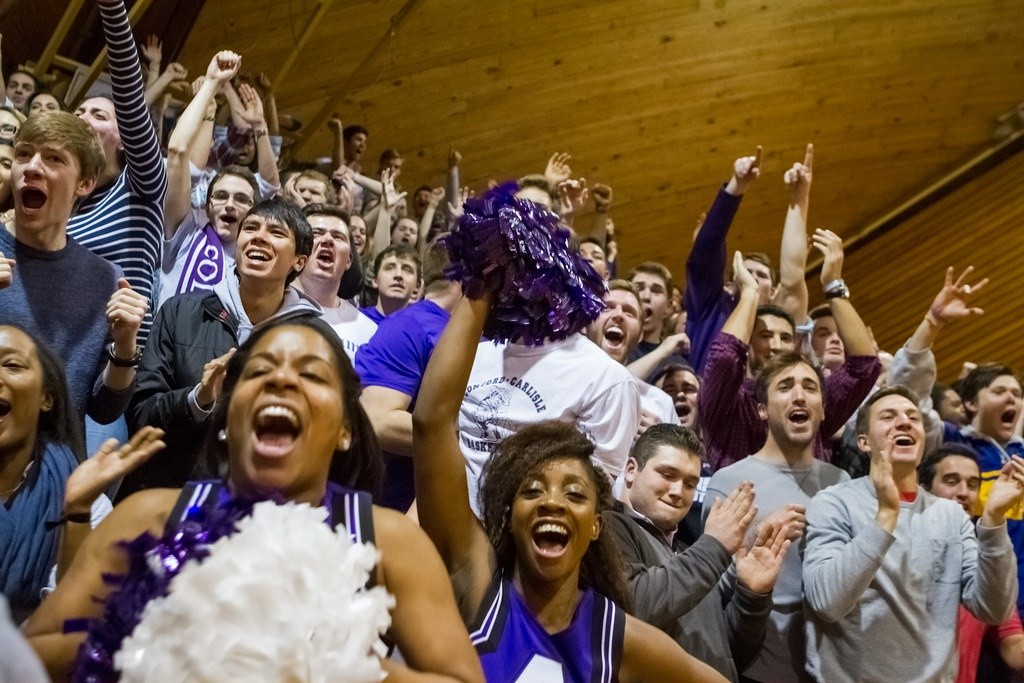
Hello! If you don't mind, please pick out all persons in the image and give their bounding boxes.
[0,0,1024,683]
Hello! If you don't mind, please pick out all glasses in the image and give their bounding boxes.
[1,124,17,135]
[211,192,254,206]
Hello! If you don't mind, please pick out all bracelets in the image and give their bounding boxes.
[203,115,215,121]
[254,129,268,135]
[823,278,844,292]
[104,341,141,367]
[44,511,91,531]
[925,313,943,330]
[57,552,77,569]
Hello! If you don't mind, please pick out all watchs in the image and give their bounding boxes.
[825,284,850,299]
